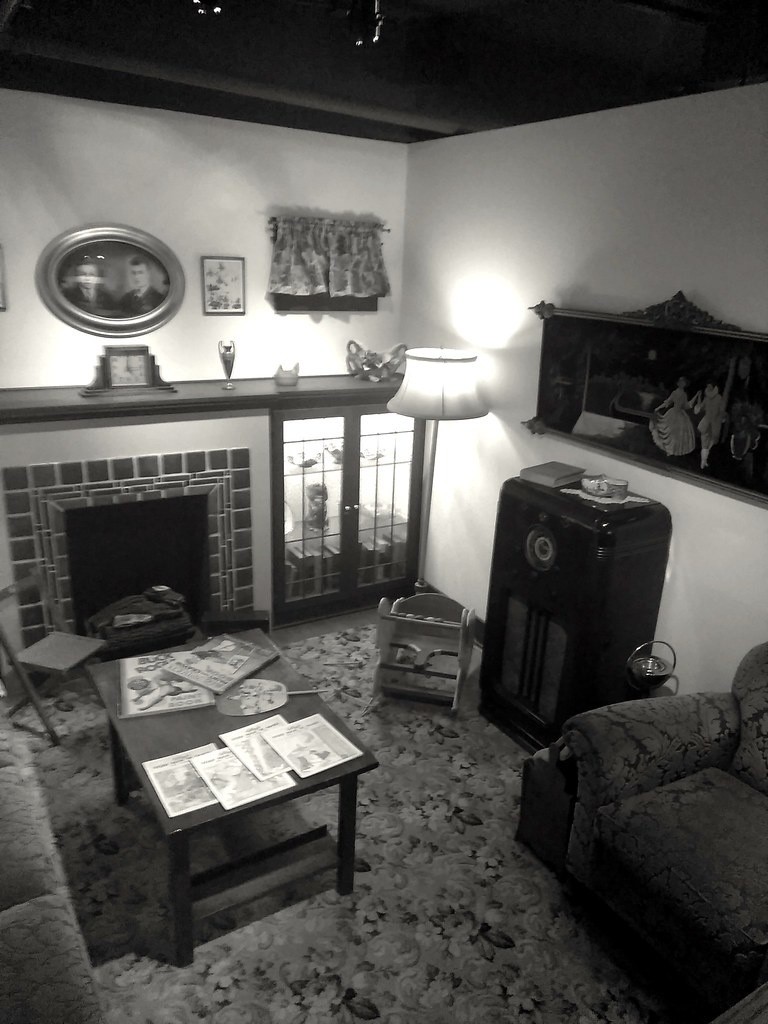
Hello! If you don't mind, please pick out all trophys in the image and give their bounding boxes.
[219,341,236,389]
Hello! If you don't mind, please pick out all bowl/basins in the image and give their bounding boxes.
[582,474,628,499]
[324,442,344,464]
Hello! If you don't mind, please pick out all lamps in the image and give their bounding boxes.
[344,0,384,45]
[386,346,490,598]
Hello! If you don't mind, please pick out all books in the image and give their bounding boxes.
[142,713,363,818]
[116,632,280,718]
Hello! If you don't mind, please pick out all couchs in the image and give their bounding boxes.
[0,742,103,1024]
[512,644,768,1024]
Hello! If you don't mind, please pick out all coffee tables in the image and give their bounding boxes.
[82,628,380,969]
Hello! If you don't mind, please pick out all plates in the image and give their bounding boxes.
[360,448,385,460]
[289,453,322,467]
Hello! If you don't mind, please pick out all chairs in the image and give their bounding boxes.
[0,567,106,745]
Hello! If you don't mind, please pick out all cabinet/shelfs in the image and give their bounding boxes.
[476,477,673,756]
[271,402,426,630]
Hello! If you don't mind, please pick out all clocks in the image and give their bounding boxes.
[79,345,180,396]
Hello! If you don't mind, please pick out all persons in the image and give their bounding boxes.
[66,256,163,318]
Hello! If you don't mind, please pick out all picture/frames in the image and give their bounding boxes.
[522,290,768,511]
[35,222,186,339]
[201,255,246,315]
